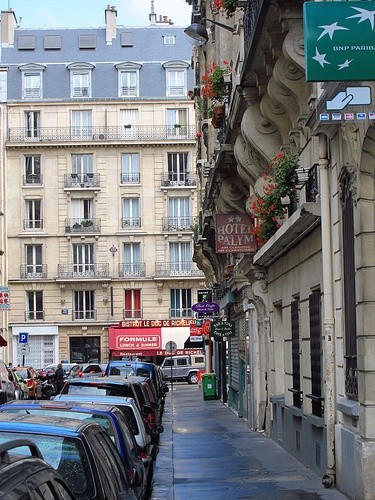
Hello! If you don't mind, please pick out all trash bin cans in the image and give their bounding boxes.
[201,373,217,401]
[198,370,206,388]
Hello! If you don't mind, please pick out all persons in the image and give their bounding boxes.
[55,364,66,394]
[7,363,12,368]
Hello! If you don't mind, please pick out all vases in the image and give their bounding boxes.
[238,0,247,7]
[280,195,291,204]
[222,73,232,82]
[294,168,309,185]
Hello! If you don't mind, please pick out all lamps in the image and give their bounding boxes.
[183,18,237,46]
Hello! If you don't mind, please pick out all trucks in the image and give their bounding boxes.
[160,355,205,384]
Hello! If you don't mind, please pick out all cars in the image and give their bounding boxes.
[0,439,80,500]
[0,361,169,500]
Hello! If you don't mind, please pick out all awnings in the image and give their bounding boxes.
[109,327,203,356]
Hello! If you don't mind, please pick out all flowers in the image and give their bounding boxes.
[200,59,228,98]
[213,0,240,19]
[249,153,297,250]
